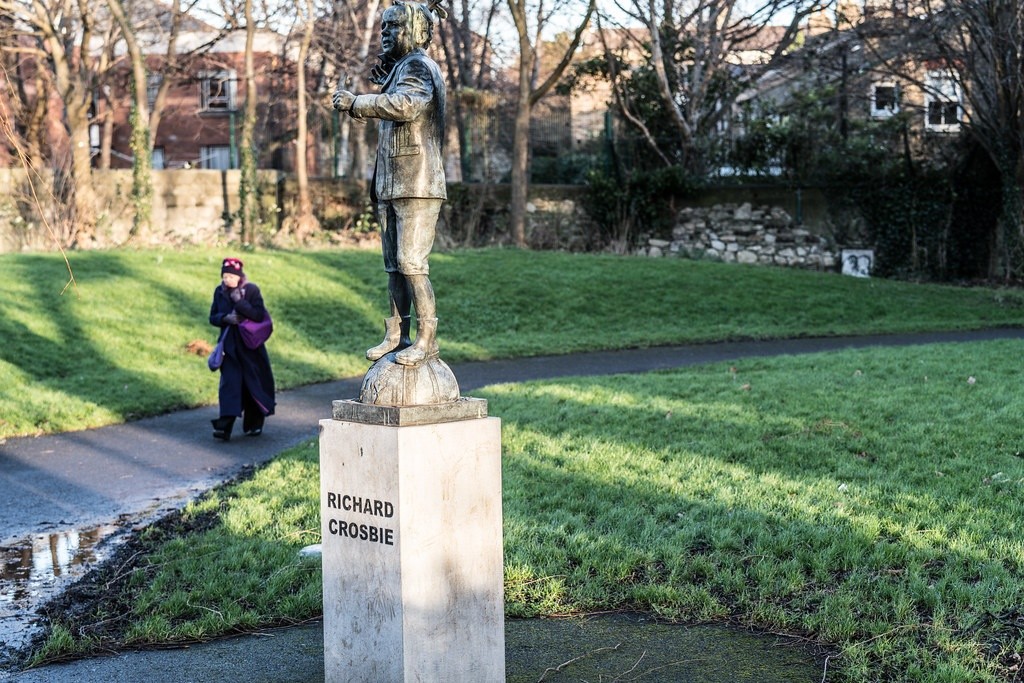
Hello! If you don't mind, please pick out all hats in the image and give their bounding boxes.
[221,258,244,276]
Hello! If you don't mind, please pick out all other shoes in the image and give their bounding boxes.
[213,431,231,441]
[246,430,262,437]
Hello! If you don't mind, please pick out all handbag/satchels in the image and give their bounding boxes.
[241,310,273,350]
[207,342,225,371]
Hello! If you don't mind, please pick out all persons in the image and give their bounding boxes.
[209,257,277,440]
[332,0,447,367]
